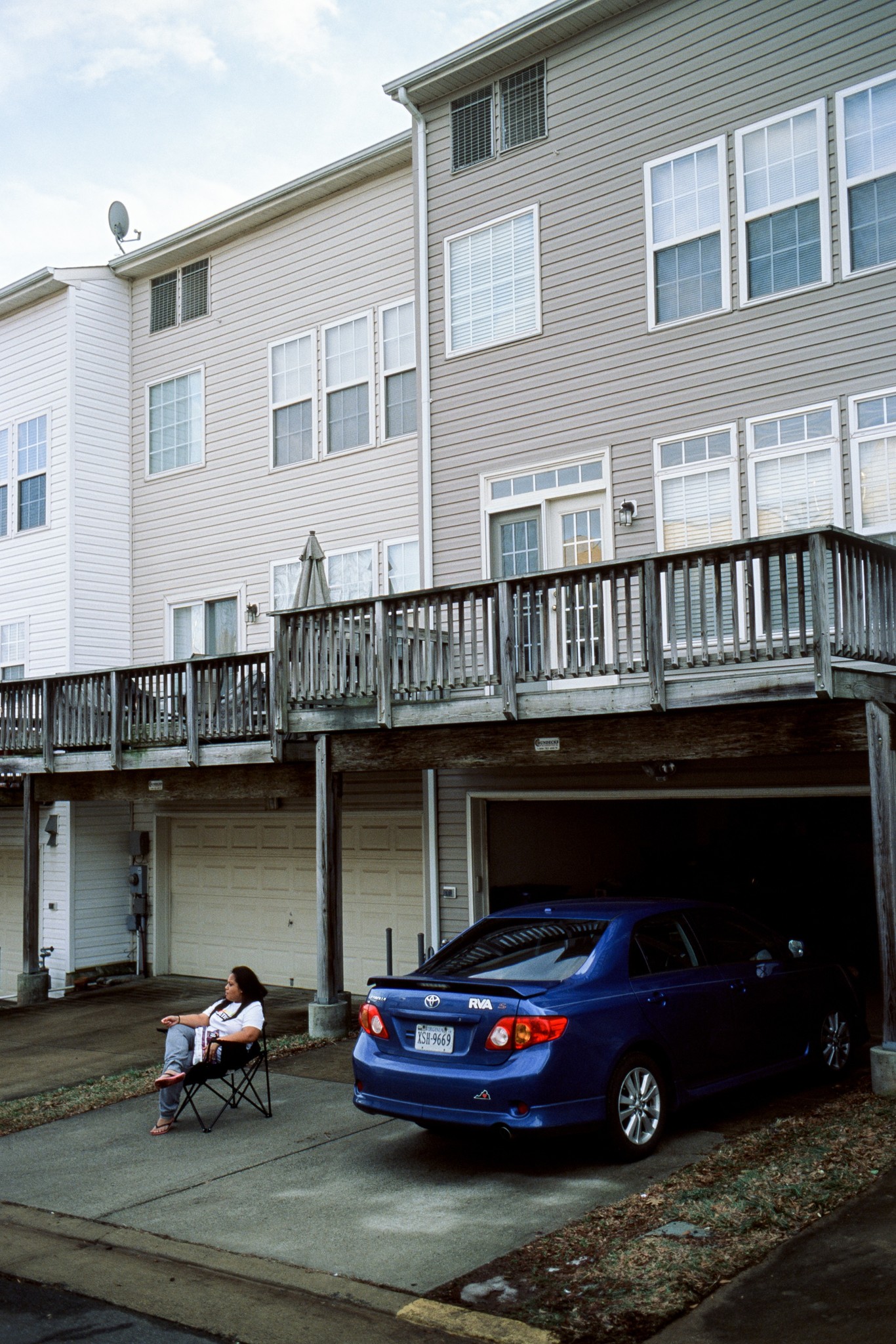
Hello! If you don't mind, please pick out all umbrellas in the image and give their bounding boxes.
[292,531,331,608]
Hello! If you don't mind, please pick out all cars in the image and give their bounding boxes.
[352,894,866,1163]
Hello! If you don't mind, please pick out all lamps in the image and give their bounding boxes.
[618,499,638,525]
[245,602,259,623]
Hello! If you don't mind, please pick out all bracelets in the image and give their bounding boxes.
[178,1015,180,1023]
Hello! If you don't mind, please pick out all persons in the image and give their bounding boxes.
[150,966,268,1135]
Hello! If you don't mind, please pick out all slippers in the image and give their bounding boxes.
[151,1116,174,1134]
[155,1070,185,1088]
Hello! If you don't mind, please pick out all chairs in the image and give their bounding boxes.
[157,1022,272,1133]
